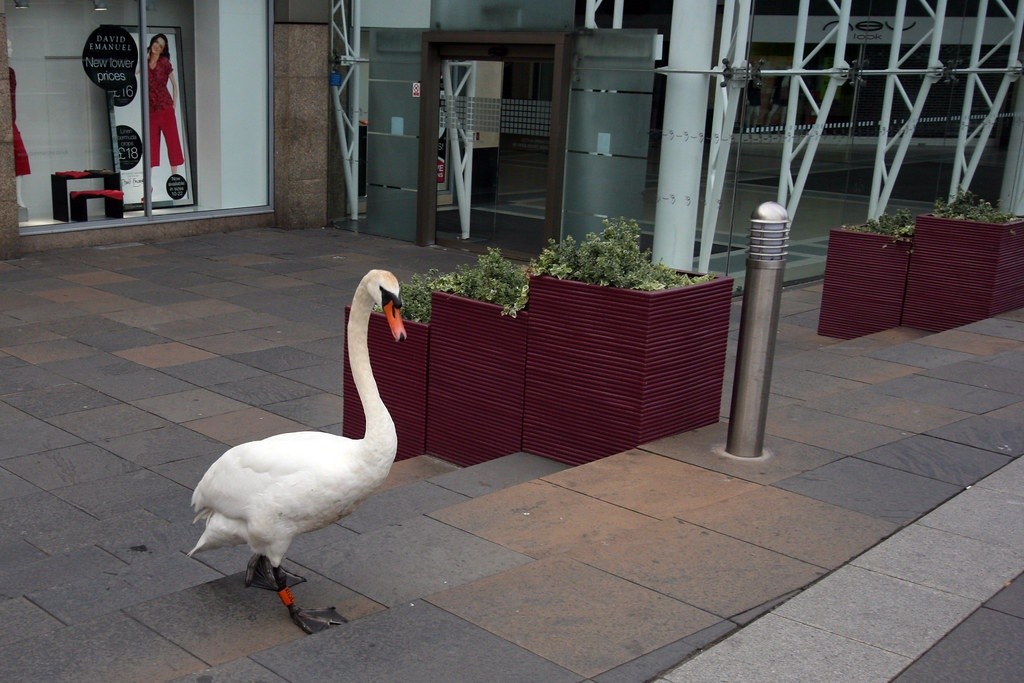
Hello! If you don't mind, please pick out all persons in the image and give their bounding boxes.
[9,66,31,210]
[141,32,185,201]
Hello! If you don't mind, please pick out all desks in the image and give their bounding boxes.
[51,173,120,222]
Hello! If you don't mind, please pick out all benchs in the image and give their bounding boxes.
[69,190,124,222]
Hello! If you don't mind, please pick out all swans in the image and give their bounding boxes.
[188,270,410,637]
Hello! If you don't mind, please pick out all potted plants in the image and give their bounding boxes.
[816,184,1024,340]
[342,215,734,467]
